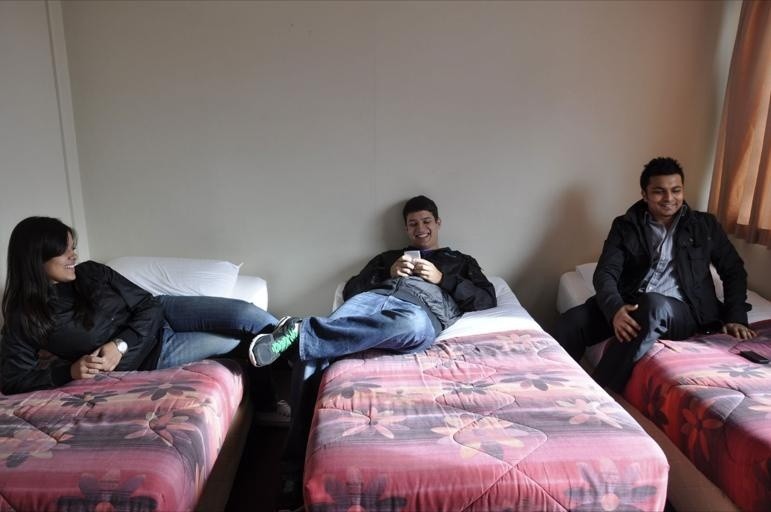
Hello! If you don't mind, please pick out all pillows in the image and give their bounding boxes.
[104,258,244,299]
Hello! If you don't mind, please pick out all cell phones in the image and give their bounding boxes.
[404,250,420,259]
[740,350,769,363]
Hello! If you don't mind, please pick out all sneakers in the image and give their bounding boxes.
[249,316,303,367]
[279,473,303,512]
[254,400,292,422]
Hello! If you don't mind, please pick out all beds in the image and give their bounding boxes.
[559,260,771,512]
[0,275,269,511]
[304,262,670,511]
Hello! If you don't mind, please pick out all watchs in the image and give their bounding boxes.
[115,338,129,355]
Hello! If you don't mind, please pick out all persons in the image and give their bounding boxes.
[248,195,498,511]
[0,216,294,427]
[552,155,760,394]
